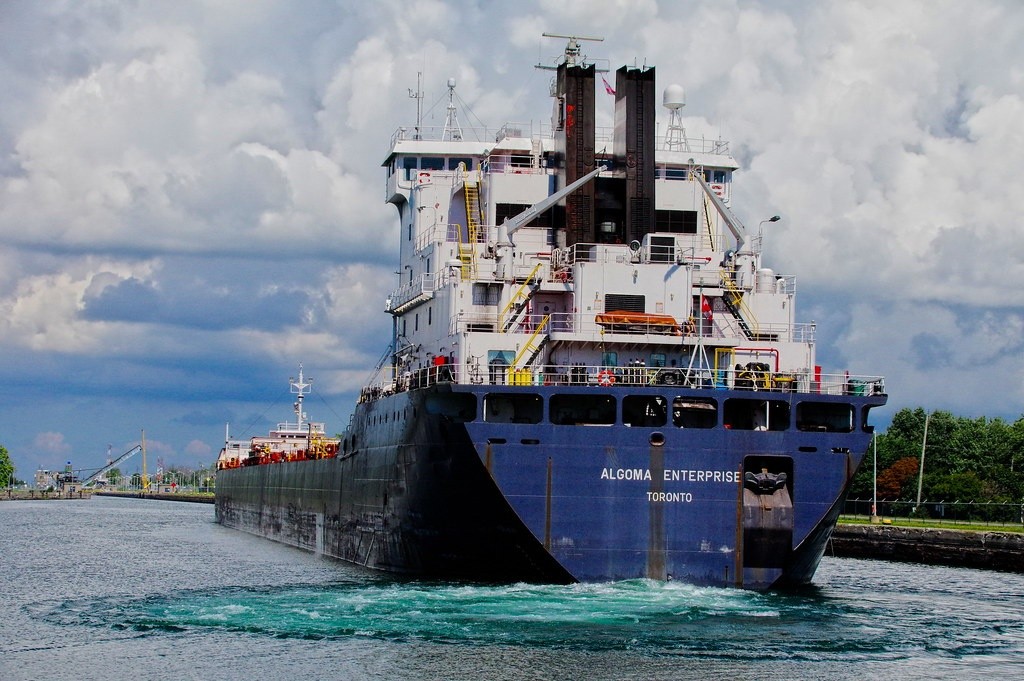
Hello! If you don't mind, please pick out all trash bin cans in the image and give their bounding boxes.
[848,379,865,396]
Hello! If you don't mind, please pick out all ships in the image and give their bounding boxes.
[214,30,892,603]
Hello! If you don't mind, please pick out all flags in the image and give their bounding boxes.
[702,294,713,325]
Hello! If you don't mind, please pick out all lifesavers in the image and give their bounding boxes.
[598,369,615,387]
[419,172,431,184]
[712,184,722,196]
